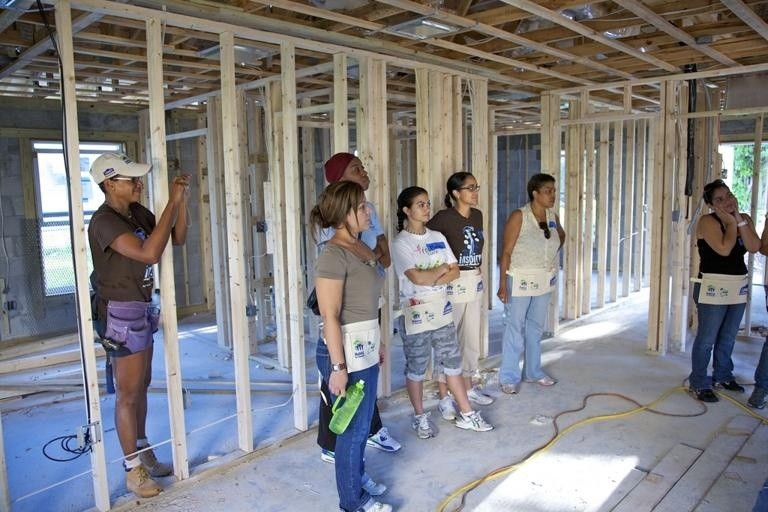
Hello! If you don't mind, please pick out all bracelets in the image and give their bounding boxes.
[737,219,749,227]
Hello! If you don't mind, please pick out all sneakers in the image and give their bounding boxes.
[689,385,717,402]
[366,427,402,452]
[126,463,161,497]
[414,385,494,439]
[355,497,392,512]
[321,449,365,463]
[362,478,386,495]
[138,445,172,476]
[712,380,744,392]
[522,375,555,386]
[747,387,768,409]
[503,384,517,394]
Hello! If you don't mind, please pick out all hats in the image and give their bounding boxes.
[325,153,354,183]
[89,151,152,184]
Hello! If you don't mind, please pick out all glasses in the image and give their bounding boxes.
[539,222,549,238]
[704,179,723,201]
[112,178,139,182]
[457,186,480,191]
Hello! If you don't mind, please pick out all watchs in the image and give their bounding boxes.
[331,363,346,371]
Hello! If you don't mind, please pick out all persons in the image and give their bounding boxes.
[746,211,768,409]
[309,180,393,512]
[393,186,494,438]
[316,152,402,463]
[688,179,761,402]
[497,173,566,395]
[87,151,193,497]
[424,172,494,420]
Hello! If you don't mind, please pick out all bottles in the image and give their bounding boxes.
[501,299,512,326]
[328,379,365,435]
